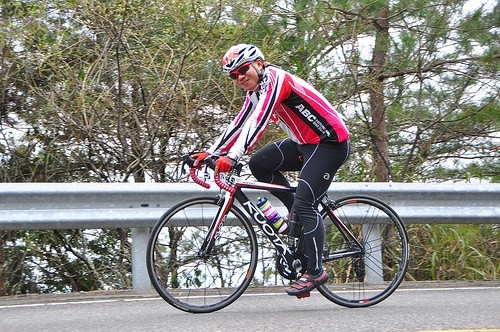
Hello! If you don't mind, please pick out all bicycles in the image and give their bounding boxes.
[147,152,410,312]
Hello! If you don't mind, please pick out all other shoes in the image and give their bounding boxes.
[285,268,328,294]
[283,213,301,236]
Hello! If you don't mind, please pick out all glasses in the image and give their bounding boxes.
[229,61,261,80]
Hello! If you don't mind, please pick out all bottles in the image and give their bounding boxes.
[256,196,288,233]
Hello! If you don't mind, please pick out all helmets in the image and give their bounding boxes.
[221,43,263,72]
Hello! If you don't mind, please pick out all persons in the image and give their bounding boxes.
[190,44,350,295]
